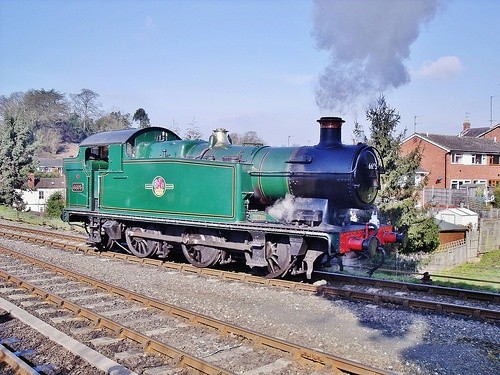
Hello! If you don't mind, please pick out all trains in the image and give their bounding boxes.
[63,116,404,280]
